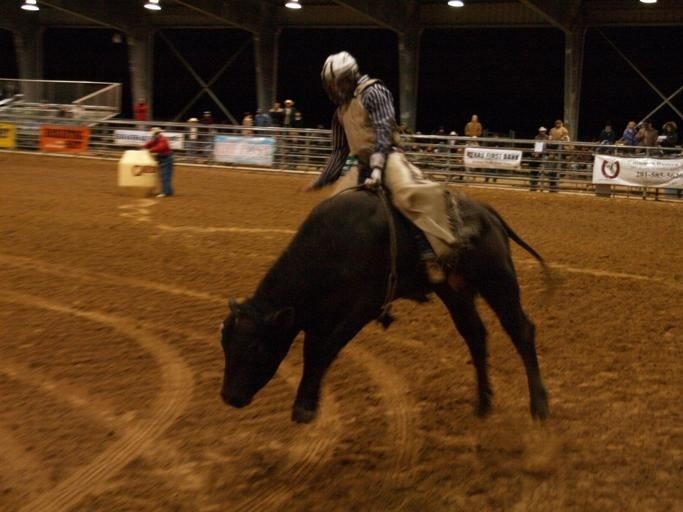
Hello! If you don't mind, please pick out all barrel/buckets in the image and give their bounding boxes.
[117,150,160,187]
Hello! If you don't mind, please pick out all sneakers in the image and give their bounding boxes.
[425,259,446,284]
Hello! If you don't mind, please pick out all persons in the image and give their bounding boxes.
[199,110,213,156]
[185,117,200,164]
[394,113,678,194]
[133,97,151,131]
[134,127,173,198]
[294,51,447,284]
[241,98,307,171]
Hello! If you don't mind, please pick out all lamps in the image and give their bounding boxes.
[19,0,40,13]
[283,0,303,11]
[143,0,162,13]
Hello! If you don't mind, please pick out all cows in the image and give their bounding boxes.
[219,186,569,426]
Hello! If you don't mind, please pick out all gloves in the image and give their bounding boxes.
[365,168,383,189]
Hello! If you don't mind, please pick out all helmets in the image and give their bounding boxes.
[320,50,360,86]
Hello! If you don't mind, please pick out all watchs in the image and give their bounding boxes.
[7,93,83,154]
